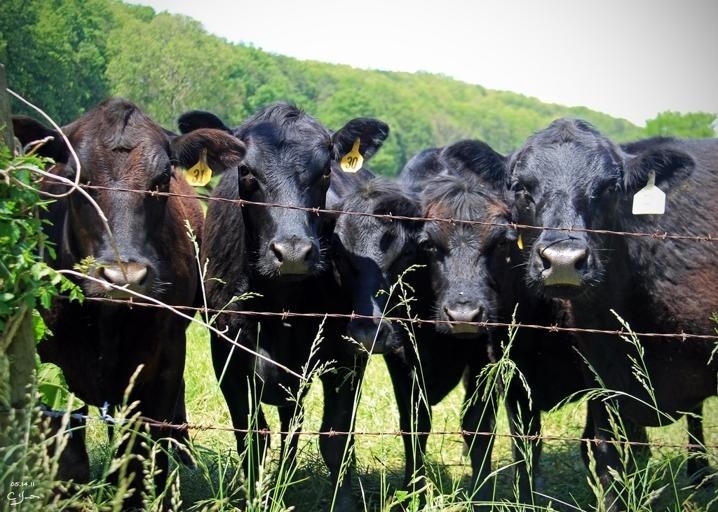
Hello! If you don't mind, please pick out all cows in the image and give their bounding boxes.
[311,174,433,512]
[434,118,718,512]
[398,146,541,512]
[10,97,248,512]
[175,100,390,512]
